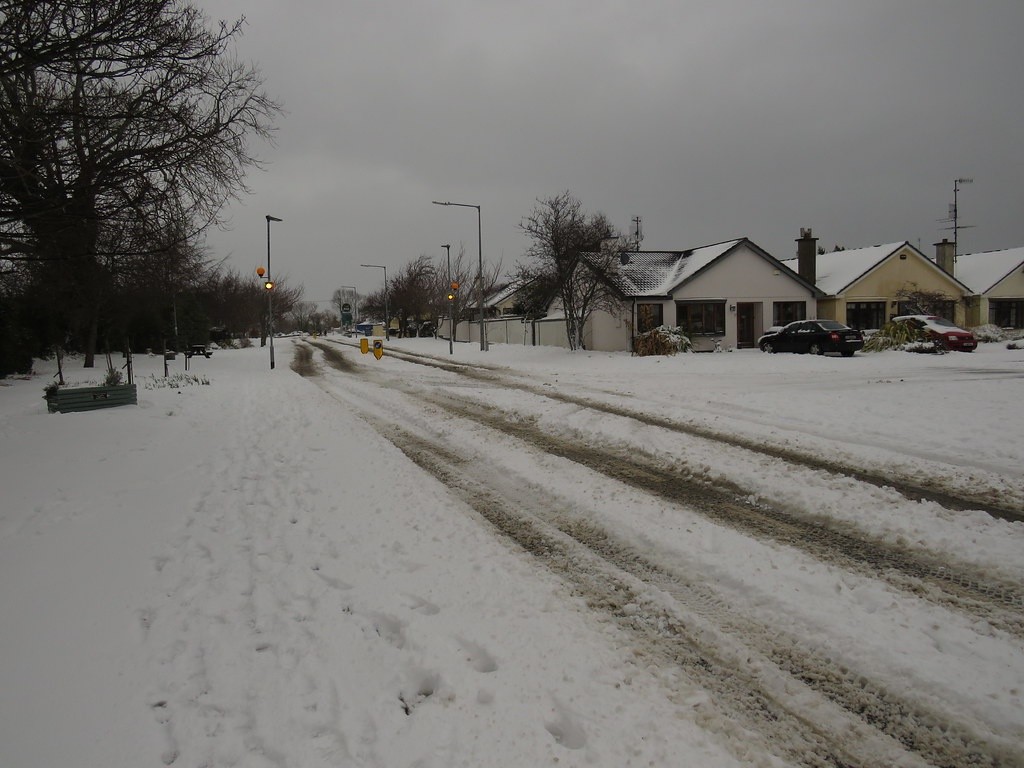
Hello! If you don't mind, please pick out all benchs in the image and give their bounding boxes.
[184,345,213,359]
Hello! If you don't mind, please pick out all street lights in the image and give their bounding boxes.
[257,215,284,370]
[361,265,389,341]
[348,287,358,338]
[431,200,486,352]
[441,244,460,353]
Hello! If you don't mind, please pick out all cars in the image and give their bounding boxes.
[757,318,864,359]
[892,314,978,352]
